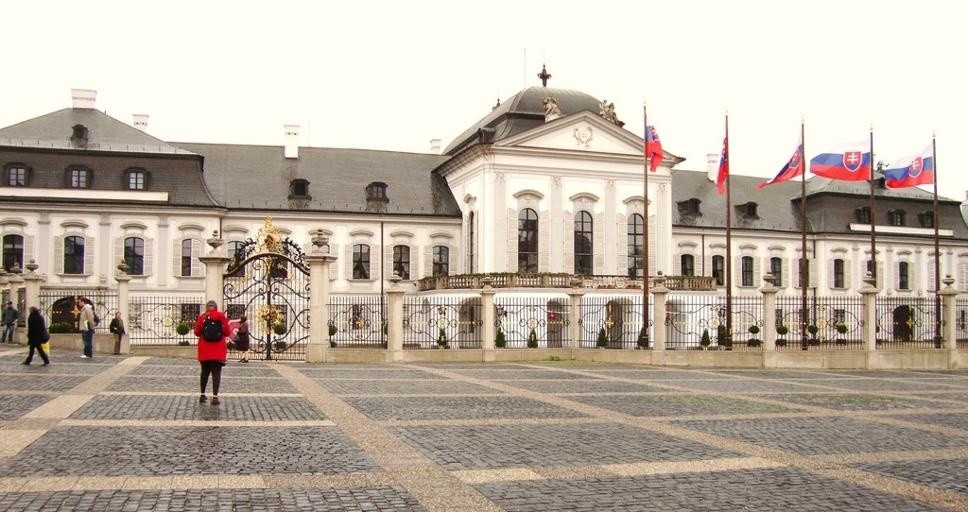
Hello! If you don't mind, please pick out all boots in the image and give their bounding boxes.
[199,395,221,405]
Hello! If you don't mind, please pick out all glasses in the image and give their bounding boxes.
[6,301,13,304]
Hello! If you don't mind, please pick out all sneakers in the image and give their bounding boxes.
[238,358,249,363]
[41,362,49,366]
[22,361,29,365]
[79,353,91,359]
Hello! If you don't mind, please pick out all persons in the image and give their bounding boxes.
[110,312,127,355]
[234,316,249,363]
[0,301,18,344]
[194,300,231,405]
[543,94,561,116]
[599,99,625,126]
[21,307,49,366]
[79,297,95,359]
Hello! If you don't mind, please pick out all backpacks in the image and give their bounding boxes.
[201,319,223,341]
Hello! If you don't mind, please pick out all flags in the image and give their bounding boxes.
[646,126,663,172]
[810,151,871,181]
[883,156,934,188]
[756,144,802,188]
[718,138,728,195]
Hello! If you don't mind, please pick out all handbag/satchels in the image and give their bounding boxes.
[39,327,49,343]
